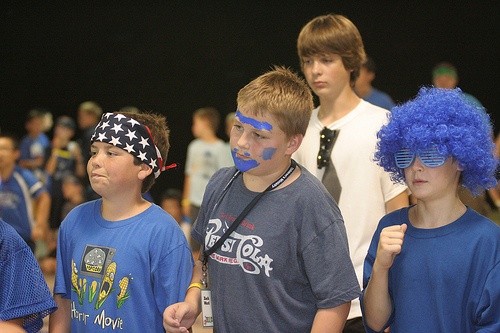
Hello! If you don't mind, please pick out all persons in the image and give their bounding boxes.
[48,113,195,333]
[1,14,500,333]
[359,85,500,333]
[1,219,58,333]
[162,66,362,333]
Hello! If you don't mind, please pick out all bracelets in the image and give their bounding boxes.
[186,282,204,290]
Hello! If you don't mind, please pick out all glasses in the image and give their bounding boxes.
[392,141,458,169]
